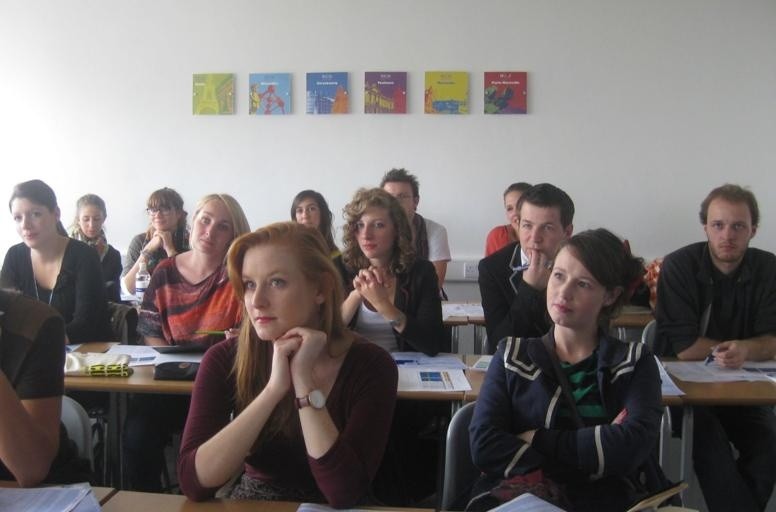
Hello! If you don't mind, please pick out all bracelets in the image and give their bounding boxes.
[139,249,151,260]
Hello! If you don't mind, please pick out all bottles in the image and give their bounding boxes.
[135,263,150,304]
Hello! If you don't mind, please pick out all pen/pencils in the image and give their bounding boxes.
[704,348,719,365]
[395,359,419,364]
[194,331,224,334]
[513,260,554,271]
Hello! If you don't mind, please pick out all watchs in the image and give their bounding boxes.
[294,389,328,410]
[386,312,405,328]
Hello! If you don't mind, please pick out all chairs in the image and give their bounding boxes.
[107,302,138,345]
[58,396,97,483]
[642,319,656,354]
[443,399,475,511]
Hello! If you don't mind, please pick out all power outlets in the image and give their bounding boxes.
[463,264,479,278]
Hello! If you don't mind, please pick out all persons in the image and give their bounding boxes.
[176,222,398,508]
[0,288,64,489]
[486,182,533,257]
[0,179,104,408]
[333,188,441,507]
[463,229,682,512]
[122,194,251,492]
[290,190,358,328]
[477,184,576,354]
[653,185,776,512]
[67,195,122,304]
[119,187,190,298]
[381,168,452,291]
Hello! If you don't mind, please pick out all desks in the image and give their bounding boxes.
[464,354,683,479]
[468,298,656,354]
[64,342,464,505]
[0,481,118,511]
[441,300,468,353]
[101,488,437,512]
[658,357,776,498]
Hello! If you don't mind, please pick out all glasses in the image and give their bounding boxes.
[147,207,178,216]
